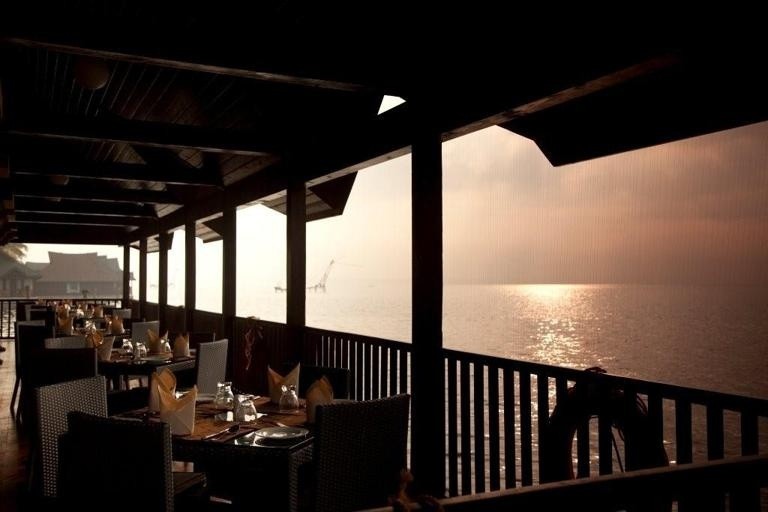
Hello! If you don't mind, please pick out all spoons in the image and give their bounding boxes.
[214,424,240,440]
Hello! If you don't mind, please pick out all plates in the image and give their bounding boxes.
[196,394,214,403]
[254,426,309,439]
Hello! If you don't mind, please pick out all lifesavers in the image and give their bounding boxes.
[540,384,669,482]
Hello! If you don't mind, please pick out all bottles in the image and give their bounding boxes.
[279,383,299,414]
[118,338,172,359]
[213,381,257,429]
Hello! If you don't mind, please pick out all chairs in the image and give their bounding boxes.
[9,298,410,510]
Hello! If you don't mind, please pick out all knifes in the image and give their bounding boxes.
[202,428,232,440]
